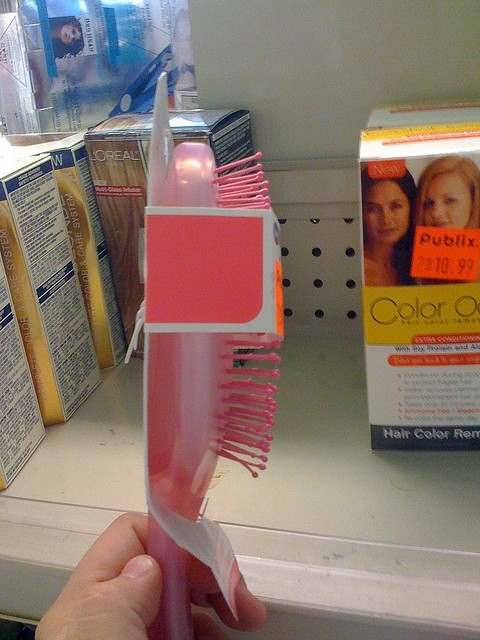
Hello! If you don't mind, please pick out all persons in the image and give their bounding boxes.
[412,156,479,232]
[360,157,419,284]
[34,509,268,640]
[46,15,85,60]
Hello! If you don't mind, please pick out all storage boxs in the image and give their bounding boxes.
[365,99,480,130]
[0,257,48,492]
[358,121,480,451]
[0,1,197,128]
[0,152,105,425]
[1,130,131,368]
[83,110,259,369]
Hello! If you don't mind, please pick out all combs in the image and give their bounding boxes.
[146,67,283,640]
